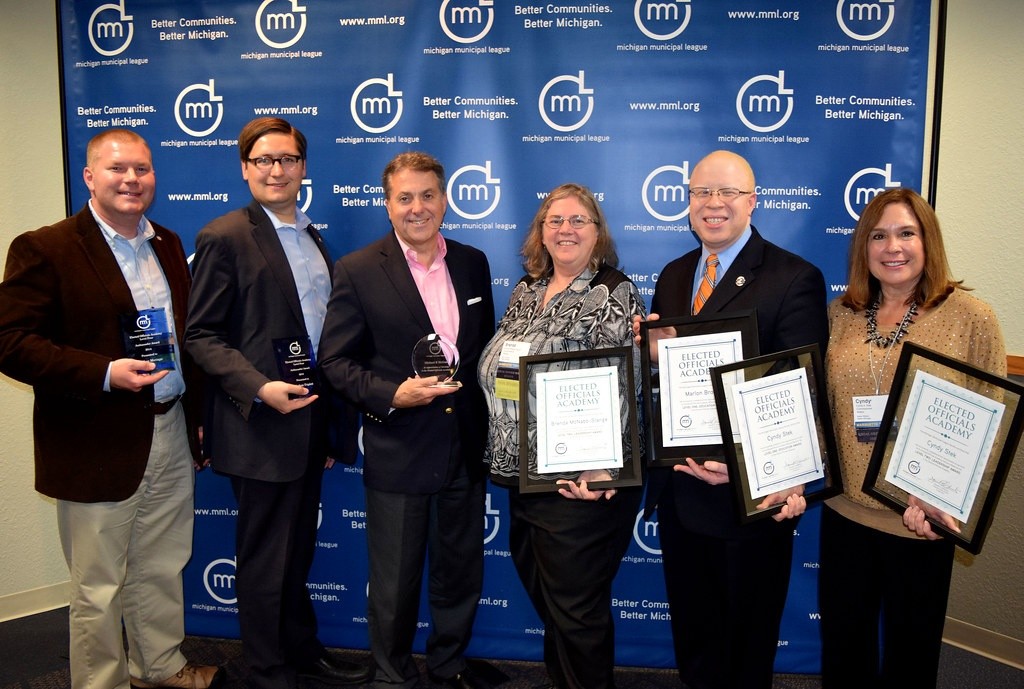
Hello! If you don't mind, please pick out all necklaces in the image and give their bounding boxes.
[868,305,912,397]
[862,289,919,350]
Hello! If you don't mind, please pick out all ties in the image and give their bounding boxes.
[693,256,719,316]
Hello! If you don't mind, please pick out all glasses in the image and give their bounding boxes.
[541,215,600,230]
[246,153,303,172]
[688,187,754,199]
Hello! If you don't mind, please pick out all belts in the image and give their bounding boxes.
[156,395,182,416]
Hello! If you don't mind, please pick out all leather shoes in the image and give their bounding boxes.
[428,662,490,689]
[294,648,373,684]
[129,663,216,689]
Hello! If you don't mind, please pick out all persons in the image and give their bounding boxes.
[632,149,833,689]
[184,116,373,689]
[316,152,500,687]
[472,182,652,689]
[0,125,220,689]
[820,181,1008,689]
